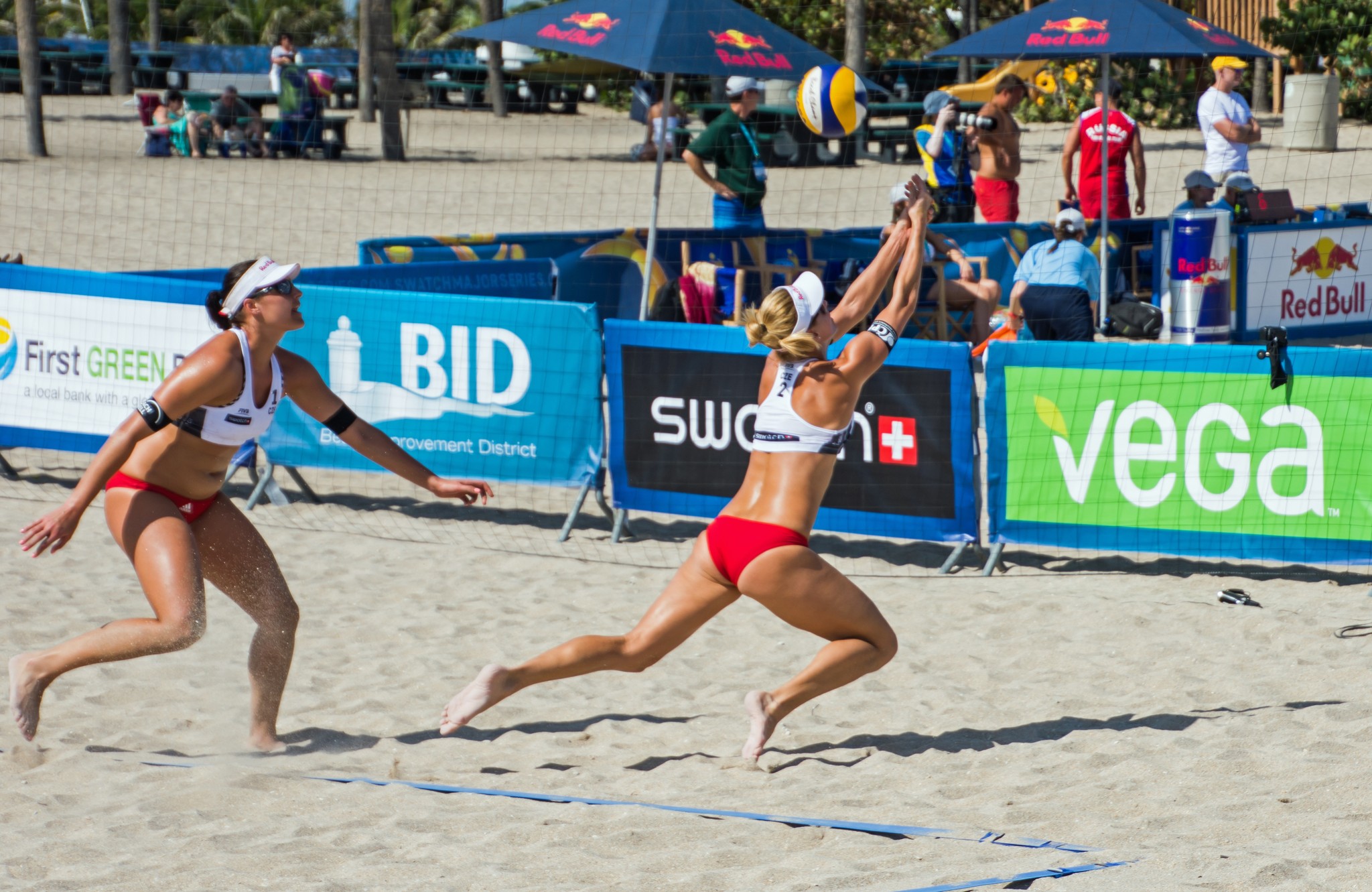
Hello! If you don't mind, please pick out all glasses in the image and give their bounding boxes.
[808,304,827,331]
[239,278,294,311]
[749,88,758,94]
[1232,68,1245,73]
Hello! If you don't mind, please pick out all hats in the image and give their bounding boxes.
[923,91,961,114]
[771,271,824,352]
[218,254,301,321]
[1225,170,1254,190]
[725,76,769,96]
[1182,170,1223,189]
[888,182,918,204]
[1054,207,1086,232]
[1211,56,1249,71]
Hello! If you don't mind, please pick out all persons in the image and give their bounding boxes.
[152,31,297,158]
[645,89,687,147]
[1197,54,1263,205]
[681,76,766,229]
[878,170,1262,342]
[437,174,941,766]
[913,90,981,224]
[973,74,1029,222]
[11,255,495,751]
[1062,78,1147,218]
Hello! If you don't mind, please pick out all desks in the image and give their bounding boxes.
[685,103,854,168]
[0,50,181,96]
[447,64,582,114]
[179,92,279,118]
[855,101,985,163]
[301,62,448,108]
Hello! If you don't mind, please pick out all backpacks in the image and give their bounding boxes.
[649,277,687,322]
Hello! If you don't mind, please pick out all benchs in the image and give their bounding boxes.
[874,130,921,163]
[329,83,378,107]
[234,115,352,145]
[79,69,114,95]
[0,68,21,93]
[134,66,195,90]
[666,128,785,168]
[424,80,521,113]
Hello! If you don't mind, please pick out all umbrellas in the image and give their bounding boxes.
[452,0,889,323]
[927,0,1277,334]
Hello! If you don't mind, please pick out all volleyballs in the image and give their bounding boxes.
[796,62,868,138]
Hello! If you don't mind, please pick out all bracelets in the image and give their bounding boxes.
[1007,312,1018,318]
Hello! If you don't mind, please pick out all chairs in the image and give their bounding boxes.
[1132,244,1153,298]
[134,92,212,158]
[682,241,772,327]
[760,237,867,333]
[884,257,989,341]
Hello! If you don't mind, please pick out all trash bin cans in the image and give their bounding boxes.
[1283,74,1342,151]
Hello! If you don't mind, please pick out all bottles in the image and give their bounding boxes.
[988,314,1007,331]
